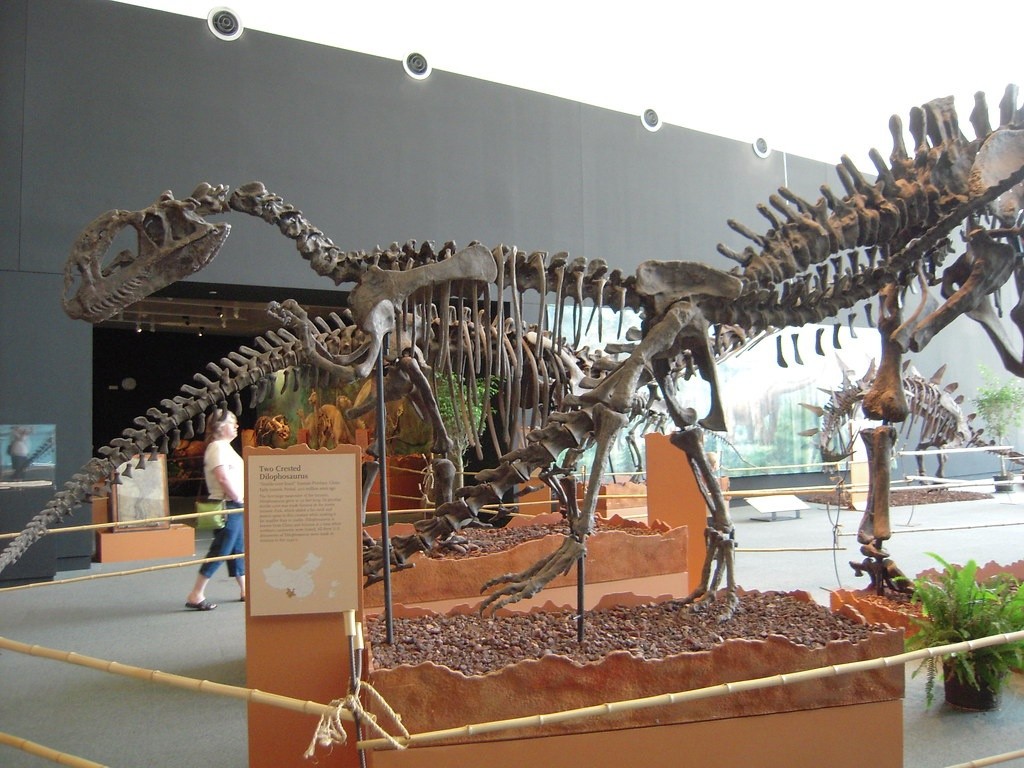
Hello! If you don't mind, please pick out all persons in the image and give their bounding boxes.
[184,409,245,611]
[698,451,720,485]
[253,416,286,448]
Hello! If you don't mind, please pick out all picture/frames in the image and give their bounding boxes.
[110,452,170,533]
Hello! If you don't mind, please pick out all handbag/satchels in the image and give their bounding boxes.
[193,501,226,531]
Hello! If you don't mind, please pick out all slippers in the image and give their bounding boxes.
[184,599,218,610]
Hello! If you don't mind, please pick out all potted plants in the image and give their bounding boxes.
[970,363,1024,492]
[891,551,1024,716]
